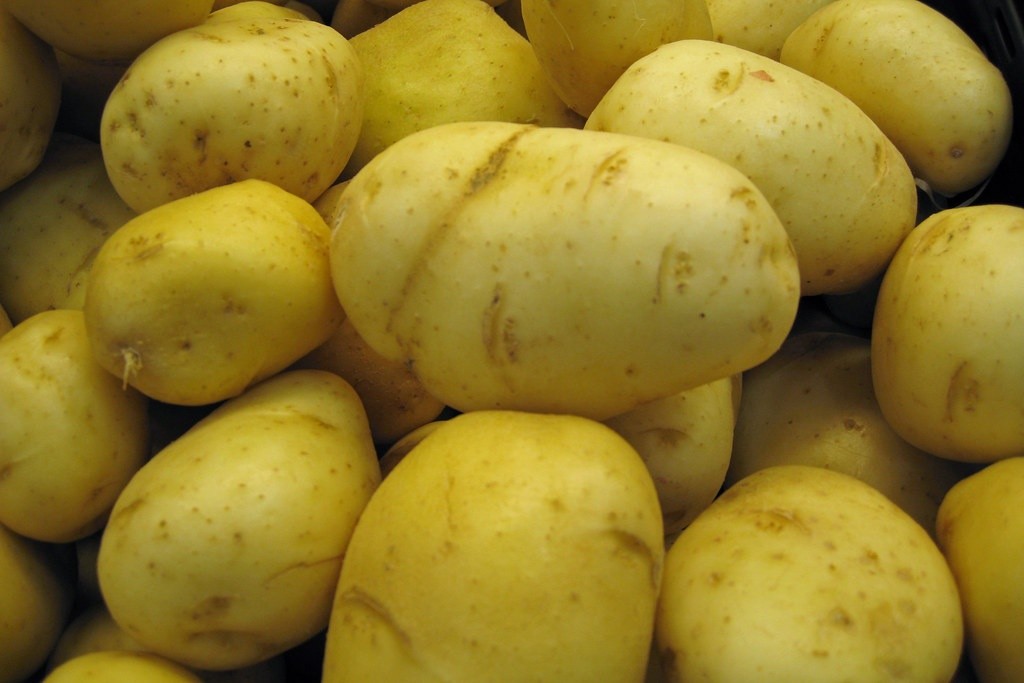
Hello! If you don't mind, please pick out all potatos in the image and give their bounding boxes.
[0,0,1024,683]
